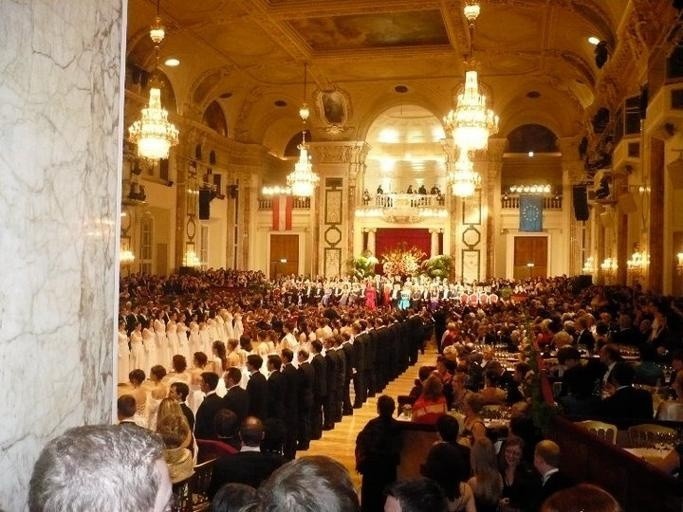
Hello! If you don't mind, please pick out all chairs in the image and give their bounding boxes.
[628,425,677,447]
[575,421,618,444]
[657,400,683,421]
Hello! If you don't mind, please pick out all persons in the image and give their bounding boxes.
[28,423,173,512]
[310,340,323,440]
[279,349,298,462]
[266,356,286,465]
[296,349,310,452]
[256,336,268,355]
[417,443,476,512]
[383,475,446,511]
[244,271,328,347]
[352,322,365,409]
[155,394,184,430]
[210,340,225,395]
[355,394,410,511]
[240,336,252,364]
[366,318,377,398]
[245,354,270,453]
[460,393,486,435]
[224,337,242,371]
[496,437,533,512]
[191,350,209,411]
[467,437,503,510]
[119,272,168,373]
[213,455,360,512]
[343,331,354,416]
[209,482,257,512]
[130,369,146,430]
[430,326,683,375]
[535,439,567,511]
[157,413,193,510]
[444,273,683,325]
[167,355,190,408]
[376,184,443,208]
[451,371,474,409]
[375,317,383,394]
[359,319,370,398]
[324,337,337,430]
[413,374,447,417]
[224,369,248,420]
[149,365,167,434]
[194,372,224,438]
[208,417,277,501]
[540,480,621,512]
[599,363,648,428]
[333,335,346,422]
[426,414,473,481]
[328,273,444,326]
[480,369,505,405]
[117,394,137,428]
[168,268,244,351]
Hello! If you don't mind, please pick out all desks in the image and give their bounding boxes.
[624,447,679,478]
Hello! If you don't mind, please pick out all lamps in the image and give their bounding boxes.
[129,0,179,160]
[442,0,500,198]
[287,65,319,201]
